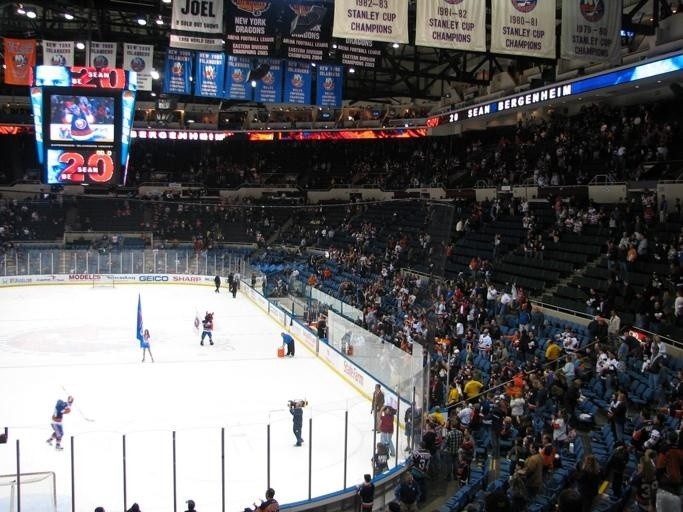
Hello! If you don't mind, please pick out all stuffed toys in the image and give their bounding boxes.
[199,311,214,346]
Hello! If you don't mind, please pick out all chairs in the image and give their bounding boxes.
[1,201,437,319]
[389,202,683,510]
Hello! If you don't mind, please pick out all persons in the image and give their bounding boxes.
[230,279,237,298]
[243,508,252,512]
[93,507,106,511]
[1,88,682,276]
[213,274,220,293]
[260,280,265,295]
[252,487,280,512]
[266,276,682,512]
[45,398,70,451]
[183,500,198,511]
[140,328,154,364]
[226,272,233,292]
[234,275,240,289]
[250,272,256,289]
[126,503,142,511]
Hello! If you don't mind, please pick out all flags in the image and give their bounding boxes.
[135,292,145,350]
